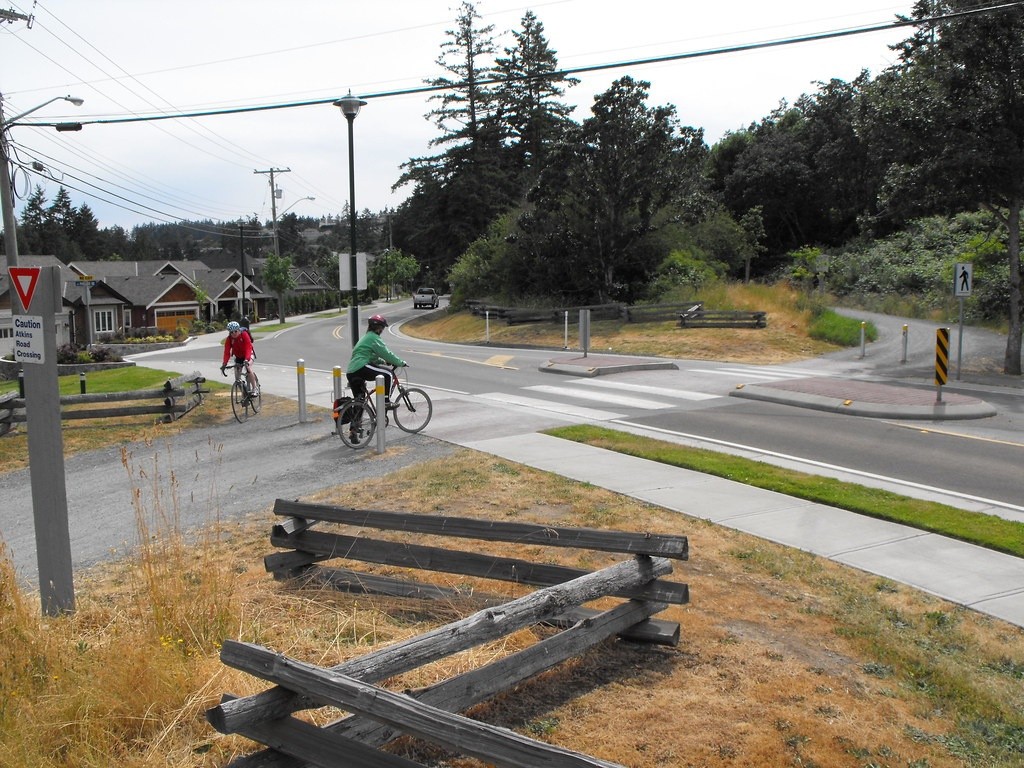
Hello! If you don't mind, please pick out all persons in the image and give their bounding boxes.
[221,321,258,402]
[240,317,249,328]
[345,316,408,444]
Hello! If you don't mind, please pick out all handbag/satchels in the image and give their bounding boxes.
[334,396,352,428]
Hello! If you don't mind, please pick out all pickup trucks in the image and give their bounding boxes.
[413,288,439,310]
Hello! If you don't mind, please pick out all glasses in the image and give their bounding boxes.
[230,331,237,333]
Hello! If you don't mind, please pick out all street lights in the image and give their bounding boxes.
[0,96,85,308]
[384,248,389,301]
[332,88,369,388]
[271,195,316,324]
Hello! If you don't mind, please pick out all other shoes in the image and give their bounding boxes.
[251,387,259,397]
[385,402,400,409]
[349,431,360,444]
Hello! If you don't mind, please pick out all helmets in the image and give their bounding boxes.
[368,315,389,327]
[226,321,240,331]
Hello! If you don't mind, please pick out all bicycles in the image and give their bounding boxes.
[334,361,431,450]
[220,360,263,424]
